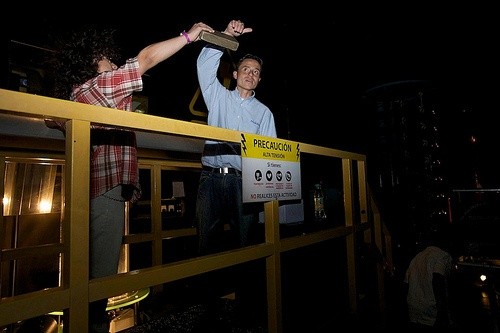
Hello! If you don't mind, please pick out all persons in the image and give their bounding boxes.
[60,22,214,333]
[404,241,463,333]
[194,19,277,333]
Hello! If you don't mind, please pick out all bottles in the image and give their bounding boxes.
[320,181,327,218]
[314,184,325,219]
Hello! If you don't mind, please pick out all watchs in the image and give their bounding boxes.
[180,30,191,44]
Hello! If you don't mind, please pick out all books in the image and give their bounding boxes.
[199,29,240,52]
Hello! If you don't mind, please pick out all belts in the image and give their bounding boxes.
[202,164,242,177]
